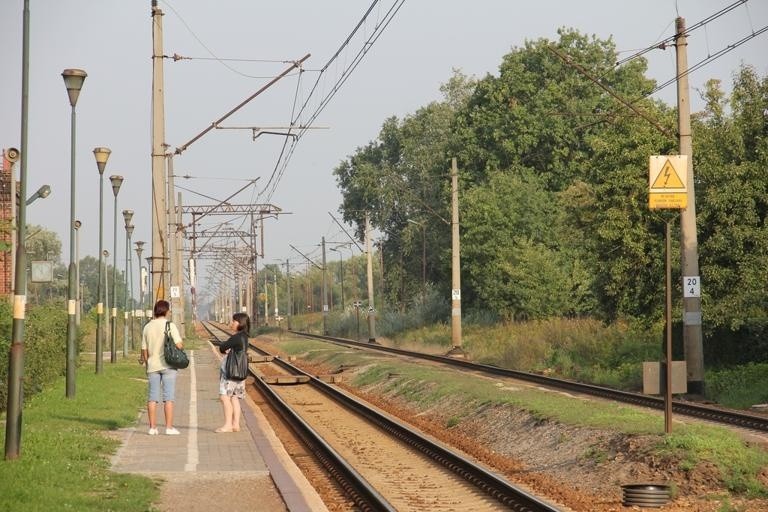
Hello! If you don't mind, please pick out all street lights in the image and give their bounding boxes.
[2,182,55,457]
[105,173,125,366]
[91,143,111,378]
[120,204,151,360]
[55,64,88,405]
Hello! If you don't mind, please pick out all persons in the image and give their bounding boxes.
[214,312,251,433]
[141,300,184,435]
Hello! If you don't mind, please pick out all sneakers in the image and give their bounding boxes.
[166,428,180,435]
[149,428,159,435]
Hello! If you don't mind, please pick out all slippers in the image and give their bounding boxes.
[215,428,233,433]
[233,429,240,432]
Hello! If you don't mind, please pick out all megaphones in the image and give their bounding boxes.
[103,249,109,256]
[74,220,81,229]
[5,147,20,164]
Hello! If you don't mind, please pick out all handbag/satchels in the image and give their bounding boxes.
[164,332,188,368]
[226,348,247,380]
